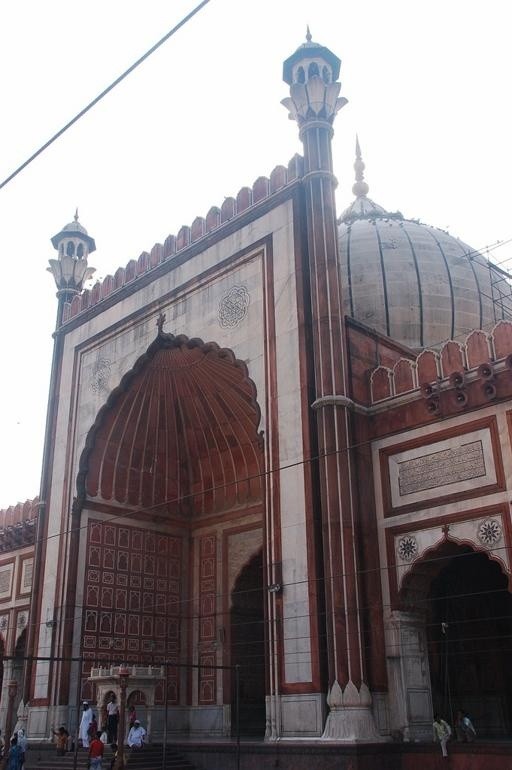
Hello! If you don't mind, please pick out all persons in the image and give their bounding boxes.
[88,731,105,770]
[78,701,93,752]
[105,743,119,769]
[127,718,147,754]
[0,728,26,770]
[127,704,136,732]
[99,725,108,744]
[106,694,121,744]
[90,712,98,734]
[453,708,477,743]
[432,712,455,759]
[50,727,68,755]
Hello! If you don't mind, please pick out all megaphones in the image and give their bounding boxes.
[479,383,498,400]
[476,363,495,383]
[505,354,512,371]
[421,383,440,399]
[422,399,440,417]
[450,390,469,408]
[448,371,468,389]
